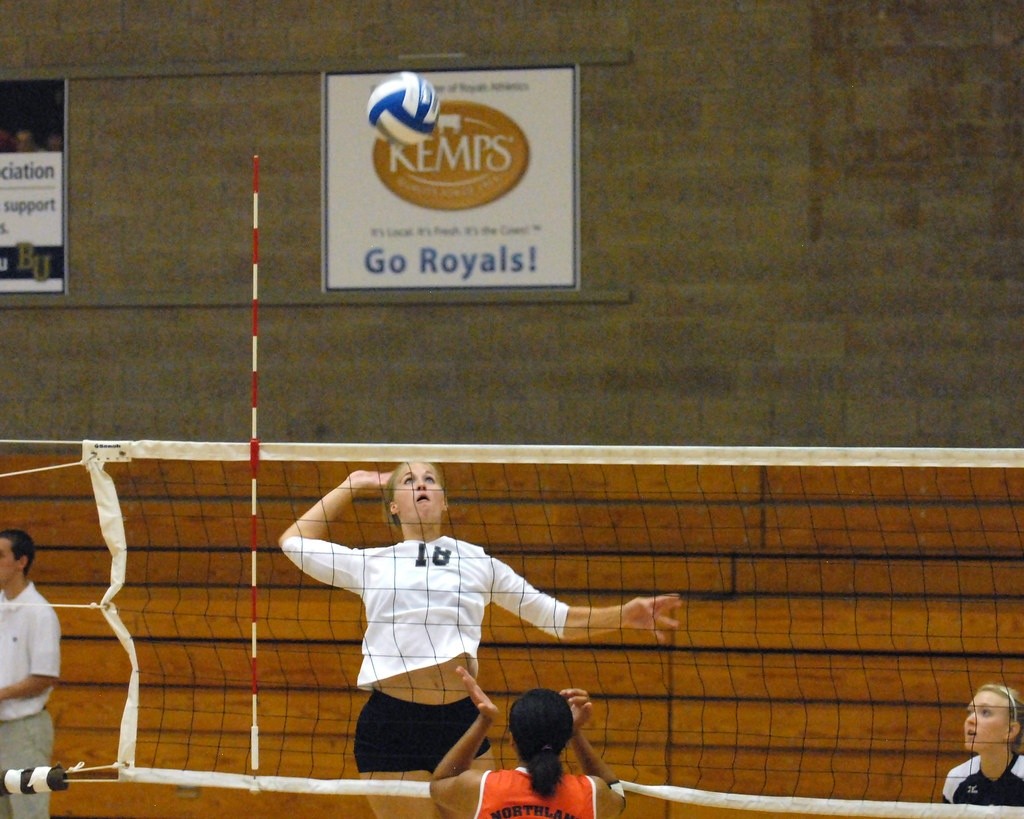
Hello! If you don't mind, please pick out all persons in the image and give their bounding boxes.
[942,684,1024,808]
[0,528,62,819]
[279,460,683,819]
[430,666,626,819]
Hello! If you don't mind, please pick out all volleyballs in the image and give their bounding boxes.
[365,71,444,147]
